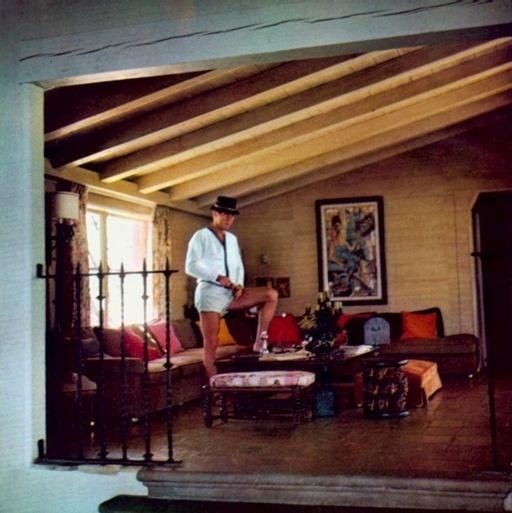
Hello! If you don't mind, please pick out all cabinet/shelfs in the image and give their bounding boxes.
[470,189,510,371]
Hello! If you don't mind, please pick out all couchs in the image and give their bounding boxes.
[297,308,480,384]
[78,307,257,419]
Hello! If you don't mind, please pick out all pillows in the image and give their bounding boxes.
[148,319,183,354]
[267,314,299,345]
[399,310,438,341]
[195,314,247,348]
[122,322,163,360]
[171,319,197,349]
[93,326,121,357]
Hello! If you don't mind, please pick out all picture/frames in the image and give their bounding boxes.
[315,196,388,306]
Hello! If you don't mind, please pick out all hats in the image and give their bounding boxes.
[210,195,240,215]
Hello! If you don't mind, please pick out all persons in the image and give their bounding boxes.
[184,195,280,403]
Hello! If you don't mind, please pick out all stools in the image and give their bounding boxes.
[201,370,315,428]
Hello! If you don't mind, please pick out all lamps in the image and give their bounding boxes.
[46,191,81,266]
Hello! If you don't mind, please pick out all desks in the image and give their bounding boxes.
[48,369,98,449]
